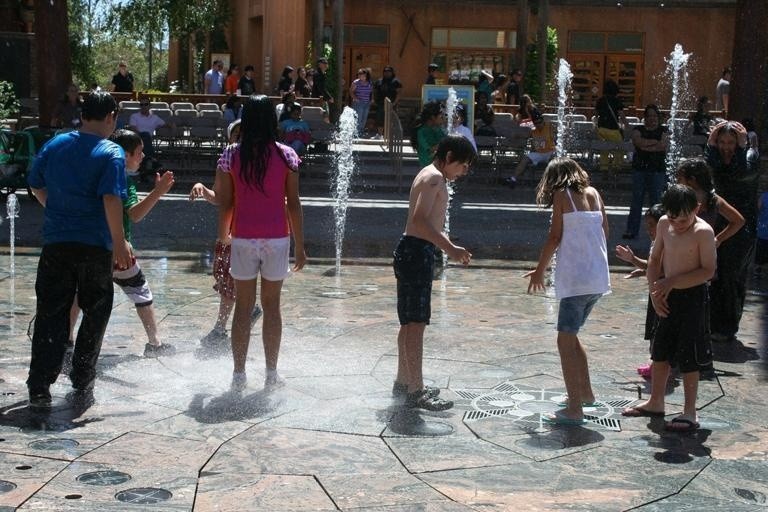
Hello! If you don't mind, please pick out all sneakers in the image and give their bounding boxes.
[250,303,263,329]
[231,377,248,393]
[264,373,285,391]
[623,231,636,239]
[29,385,52,413]
[371,134,384,139]
[200,329,228,348]
[711,331,728,342]
[637,365,652,377]
[498,177,516,189]
[393,380,440,398]
[71,391,95,416]
[405,385,453,411]
[143,341,176,359]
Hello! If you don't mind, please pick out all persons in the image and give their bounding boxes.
[190,119,263,348]
[26,91,136,411]
[64,128,175,357]
[204,59,403,159]
[111,64,135,103]
[594,79,631,180]
[411,61,559,188]
[49,86,84,134]
[214,93,306,394]
[392,134,477,410]
[90,84,101,93]
[616,68,767,432]
[522,156,611,425]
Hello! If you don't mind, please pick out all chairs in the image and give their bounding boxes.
[474,112,708,170]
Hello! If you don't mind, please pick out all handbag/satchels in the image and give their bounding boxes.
[287,131,310,146]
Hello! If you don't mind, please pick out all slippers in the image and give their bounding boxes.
[665,416,699,432]
[621,404,665,418]
[557,398,598,408]
[542,410,588,425]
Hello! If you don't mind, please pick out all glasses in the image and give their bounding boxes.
[139,103,149,107]
